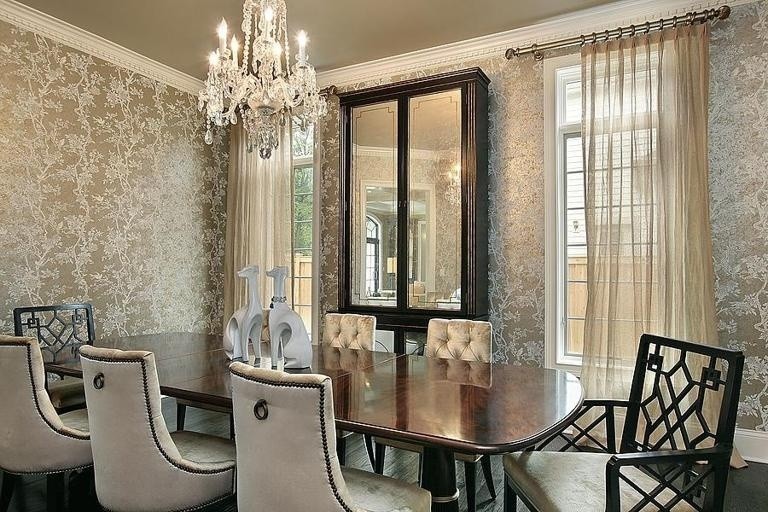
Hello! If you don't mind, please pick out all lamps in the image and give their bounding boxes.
[196,0,327,160]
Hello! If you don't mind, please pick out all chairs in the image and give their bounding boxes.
[12,304,97,415]
[376,318,496,510]
[502,334,743,512]
[228,361,432,510]
[321,313,376,471]
[230,309,272,442]
[80,345,237,509]
[0,335,92,509]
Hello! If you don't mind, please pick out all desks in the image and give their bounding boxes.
[36,332,585,510]
